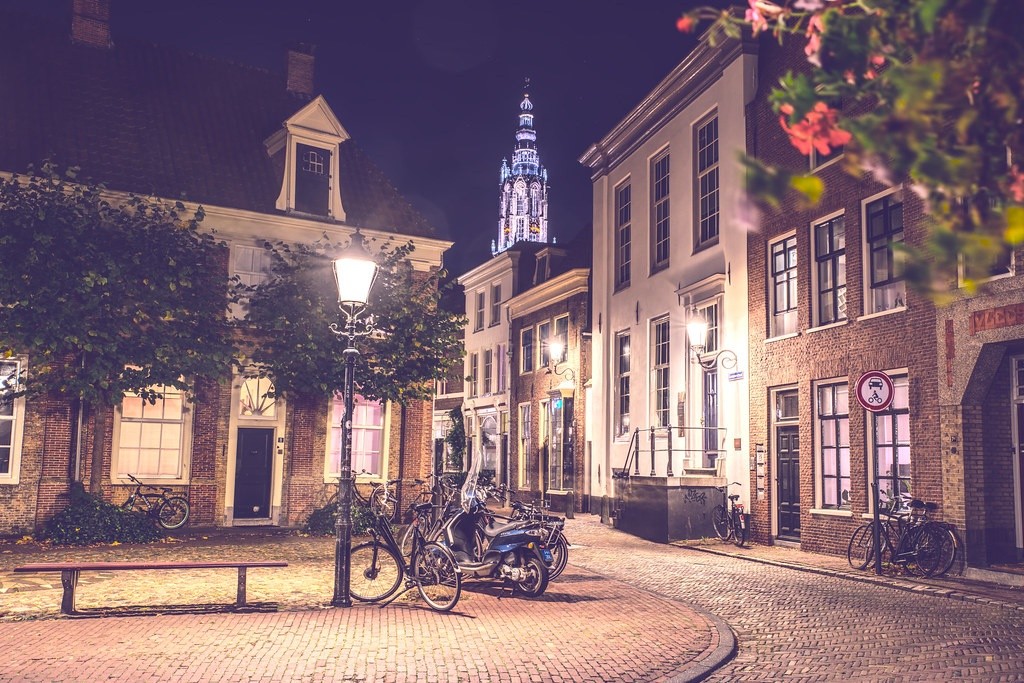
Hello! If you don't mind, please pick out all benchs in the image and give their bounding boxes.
[12,559,290,613]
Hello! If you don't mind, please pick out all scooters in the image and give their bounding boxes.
[433,502,556,599]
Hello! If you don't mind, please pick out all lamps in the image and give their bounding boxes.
[548,337,575,385]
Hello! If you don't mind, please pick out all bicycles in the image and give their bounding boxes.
[848,489,957,579]
[120,473,190,529]
[711,482,746,547]
[400,472,571,581]
[349,479,463,611]
[329,468,397,526]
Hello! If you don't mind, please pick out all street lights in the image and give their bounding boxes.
[330,225,380,607]
